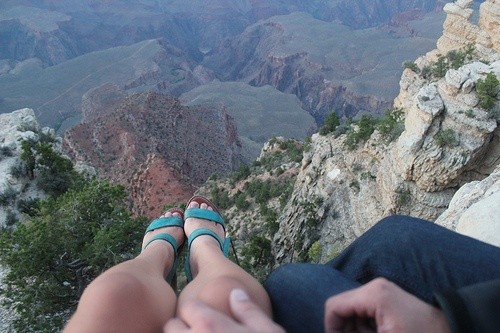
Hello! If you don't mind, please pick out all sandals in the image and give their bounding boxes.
[141,207,187,292]
[183,195,230,284]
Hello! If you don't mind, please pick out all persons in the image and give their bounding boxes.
[63,195,273,333]
[165,215,500,332]
